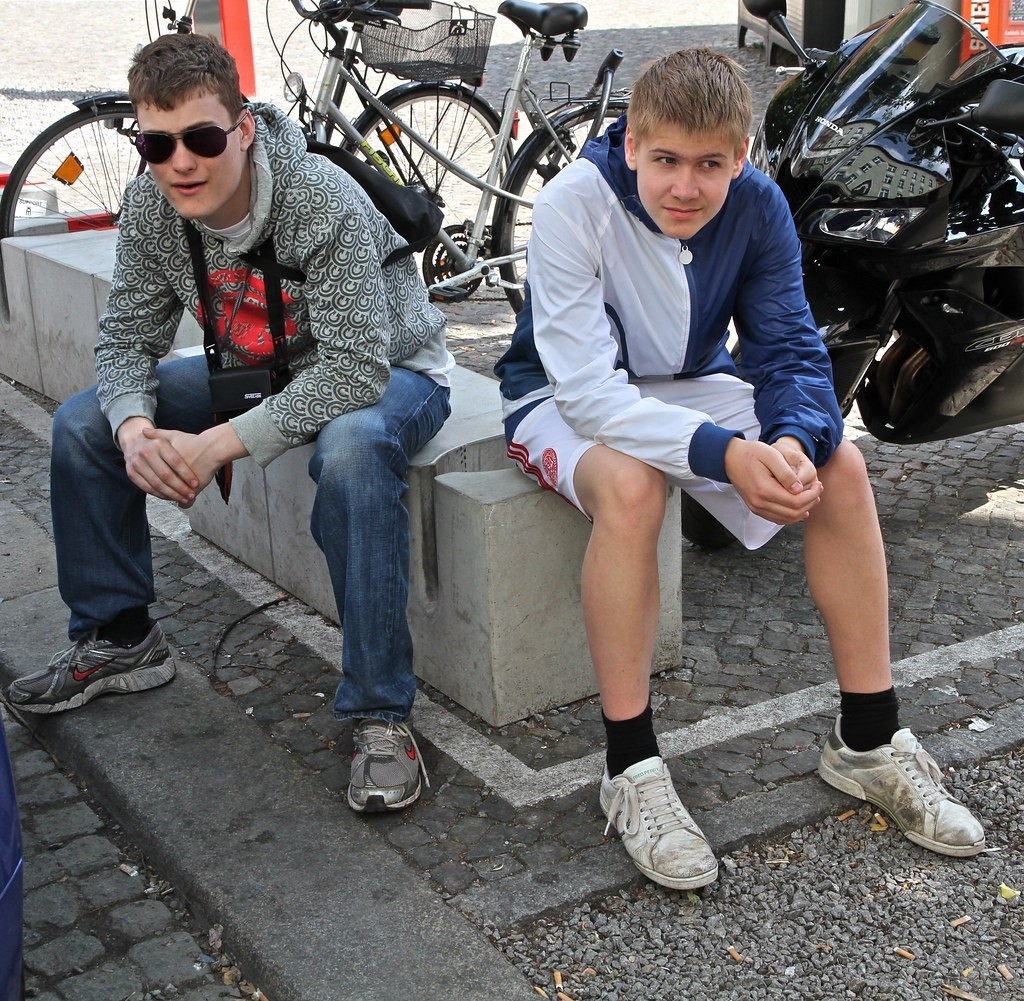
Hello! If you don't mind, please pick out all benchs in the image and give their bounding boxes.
[0,233,683,728]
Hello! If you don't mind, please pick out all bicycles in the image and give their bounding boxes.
[2,0,629,322]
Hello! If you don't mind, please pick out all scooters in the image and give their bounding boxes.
[681,0,1024,552]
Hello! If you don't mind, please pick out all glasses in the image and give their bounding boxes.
[127,106,247,164]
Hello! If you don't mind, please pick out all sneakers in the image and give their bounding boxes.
[347,709,431,811]
[599,757,719,889]
[6,621,176,714]
[819,714,985,857]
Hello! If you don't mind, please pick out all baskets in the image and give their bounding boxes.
[360,0,497,82]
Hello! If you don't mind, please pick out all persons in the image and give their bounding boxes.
[8,33,455,815]
[494,48,986,889]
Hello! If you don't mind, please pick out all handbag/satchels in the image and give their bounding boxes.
[303,132,445,267]
[208,363,291,426]
